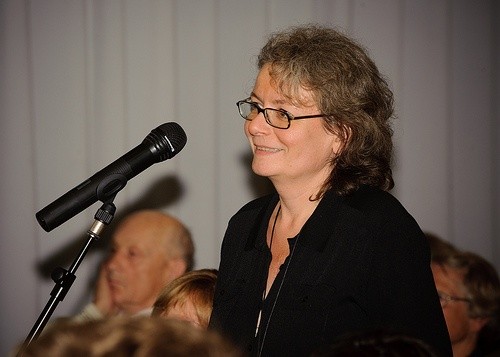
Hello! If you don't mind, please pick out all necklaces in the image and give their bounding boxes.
[255,203,300,357]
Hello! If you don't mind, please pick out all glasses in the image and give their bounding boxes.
[236,97,333,129]
[438,290,473,308]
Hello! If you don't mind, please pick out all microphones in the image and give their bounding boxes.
[36,122,187,232]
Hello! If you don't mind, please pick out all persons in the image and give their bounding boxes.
[21,208,500,357]
[201,25,454,357]
